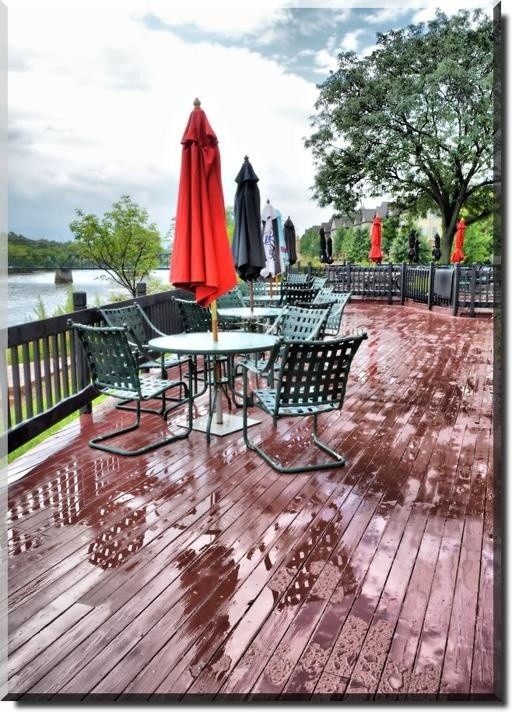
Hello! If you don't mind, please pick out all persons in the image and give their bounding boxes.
[229,155,266,311]
[169,97,238,339]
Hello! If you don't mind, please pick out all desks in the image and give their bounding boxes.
[145,331,281,437]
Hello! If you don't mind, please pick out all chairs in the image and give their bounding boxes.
[240,331,369,472]
[88,476,363,609]
[67,318,194,458]
[94,299,196,418]
[171,272,354,405]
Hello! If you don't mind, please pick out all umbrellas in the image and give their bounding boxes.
[406,227,418,258]
[324,234,334,264]
[449,217,465,262]
[259,198,287,293]
[283,216,298,265]
[413,239,418,263]
[318,227,328,263]
[276,208,291,265]
[430,232,441,260]
[367,211,381,262]
